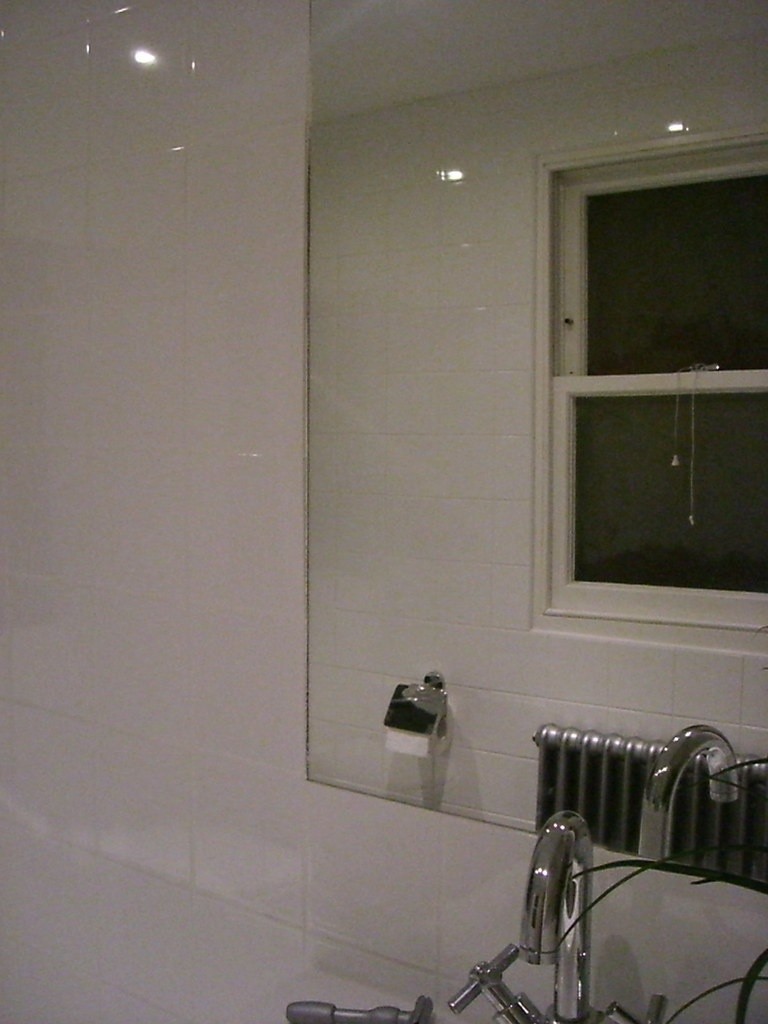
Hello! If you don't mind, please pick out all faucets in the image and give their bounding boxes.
[638,724,739,860]
[517,809,602,1024]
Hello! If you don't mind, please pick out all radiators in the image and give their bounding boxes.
[532,723,768,886]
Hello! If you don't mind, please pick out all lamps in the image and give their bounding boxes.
[383,672,447,756]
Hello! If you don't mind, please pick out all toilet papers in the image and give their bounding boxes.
[383,701,454,759]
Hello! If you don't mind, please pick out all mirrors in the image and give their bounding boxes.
[304,23,768,896]
